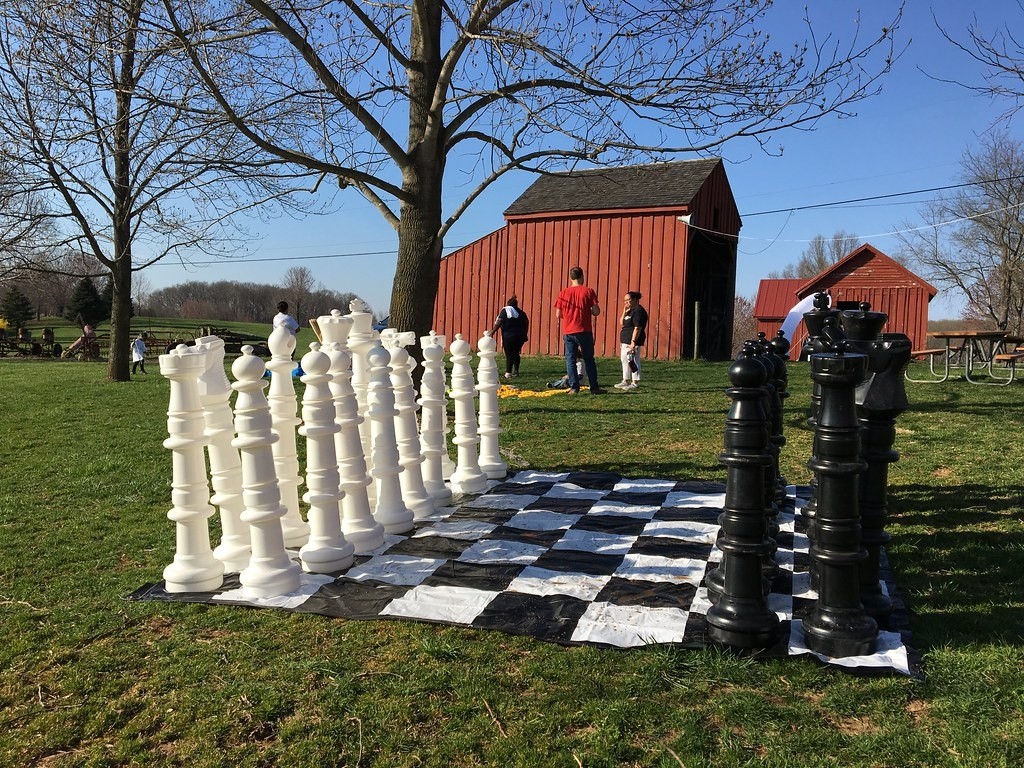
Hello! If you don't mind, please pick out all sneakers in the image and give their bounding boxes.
[614,382,629,388]
[624,384,639,391]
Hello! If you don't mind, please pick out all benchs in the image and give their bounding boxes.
[948,346,981,369]
[991,351,1024,387]
[1015,347,1024,371]
[904,349,946,382]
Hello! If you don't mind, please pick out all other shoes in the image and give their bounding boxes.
[500,374,512,379]
[566,389,579,395]
[511,371,519,377]
[592,388,607,394]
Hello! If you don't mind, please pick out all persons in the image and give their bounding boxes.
[488,294,529,380]
[554,267,608,395]
[272,302,301,361]
[130,332,149,376]
[165,339,184,355]
[613,292,649,390]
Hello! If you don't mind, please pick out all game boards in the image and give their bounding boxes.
[121,470,924,680]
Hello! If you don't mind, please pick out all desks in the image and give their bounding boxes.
[957,335,1024,369]
[926,330,1013,382]
